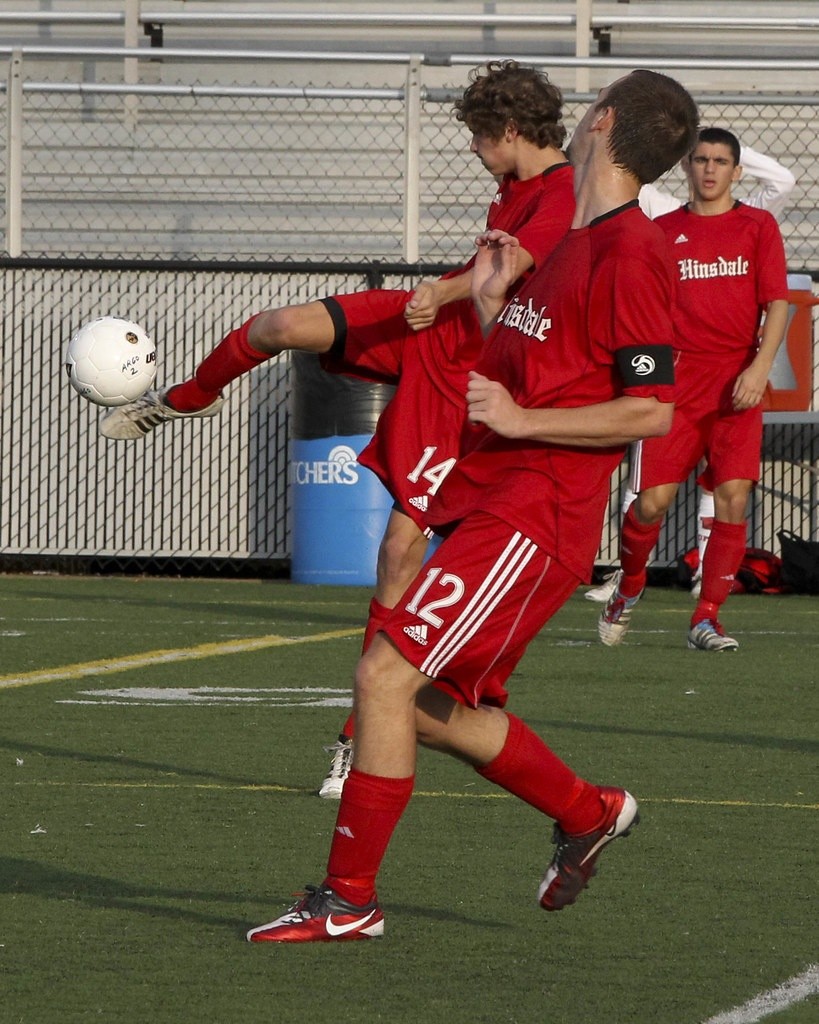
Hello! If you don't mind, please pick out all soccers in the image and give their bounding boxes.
[64,316,157,406]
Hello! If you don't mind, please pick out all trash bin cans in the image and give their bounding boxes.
[287,345,446,588]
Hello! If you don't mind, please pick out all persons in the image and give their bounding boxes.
[596,126,792,653]
[96,59,799,946]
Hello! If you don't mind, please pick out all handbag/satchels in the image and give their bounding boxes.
[777,530,819,596]
[675,546,781,594]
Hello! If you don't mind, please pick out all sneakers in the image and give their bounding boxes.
[691,569,703,600]
[537,784,641,912]
[318,734,354,800]
[247,885,385,943]
[584,568,624,603]
[598,585,646,646]
[100,382,224,440]
[687,620,739,652]
